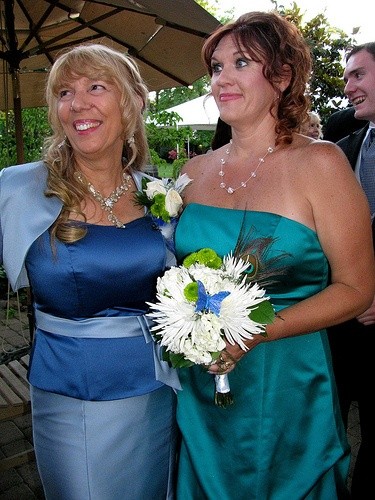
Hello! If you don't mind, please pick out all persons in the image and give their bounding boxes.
[304,41,375,500]
[0,43,180,500]
[146,10,375,499]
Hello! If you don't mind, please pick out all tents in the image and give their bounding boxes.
[147,91,220,159]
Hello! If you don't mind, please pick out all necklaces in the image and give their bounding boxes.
[71,168,133,229]
[219,139,278,195]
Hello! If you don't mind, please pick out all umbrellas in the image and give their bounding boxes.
[0,0,224,167]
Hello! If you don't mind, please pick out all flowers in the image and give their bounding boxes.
[130,172,196,232]
[143,247,287,410]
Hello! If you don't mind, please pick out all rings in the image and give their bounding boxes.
[225,360,231,370]
[219,355,225,364]
